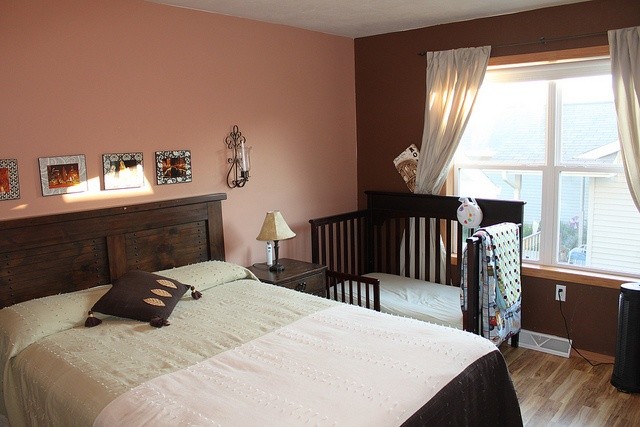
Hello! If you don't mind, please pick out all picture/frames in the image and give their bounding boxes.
[155,149,192,185]
[0,159,21,201]
[38,154,88,197]
[102,152,145,190]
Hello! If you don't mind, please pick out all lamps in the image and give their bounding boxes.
[226,124,252,189]
[256,212,296,271]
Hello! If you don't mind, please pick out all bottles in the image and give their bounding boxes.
[266,241,273,266]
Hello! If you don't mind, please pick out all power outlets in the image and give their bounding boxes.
[555,284,566,302]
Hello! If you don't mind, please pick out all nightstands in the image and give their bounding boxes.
[245,258,328,301]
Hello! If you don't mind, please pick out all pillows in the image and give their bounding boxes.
[85,267,202,328]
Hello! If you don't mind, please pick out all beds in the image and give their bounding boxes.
[1,191,524,426]
[309,191,527,350]
